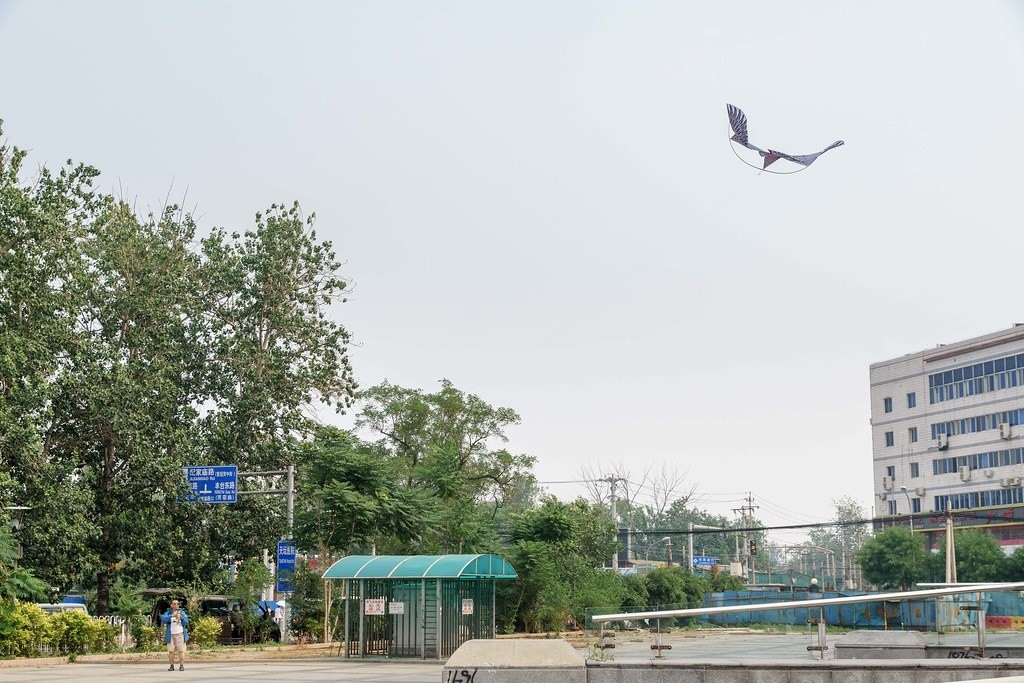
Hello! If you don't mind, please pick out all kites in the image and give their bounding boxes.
[727,103,845,174]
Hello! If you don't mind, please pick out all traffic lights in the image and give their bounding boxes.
[750,540,756,555]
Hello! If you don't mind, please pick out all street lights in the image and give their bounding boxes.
[645,536,670,575]
[900,486,915,569]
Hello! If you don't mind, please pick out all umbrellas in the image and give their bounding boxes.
[275,601,285,608]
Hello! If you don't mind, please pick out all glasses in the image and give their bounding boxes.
[172,603,180,605]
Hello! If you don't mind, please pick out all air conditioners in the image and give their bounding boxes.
[1010,476,1020,487]
[999,423,1010,438]
[915,487,924,496]
[882,476,892,490]
[985,469,991,478]
[937,435,946,447]
[959,464,969,482]
[999,477,1006,488]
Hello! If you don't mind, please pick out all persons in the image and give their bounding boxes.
[264,606,283,618]
[161,600,189,671]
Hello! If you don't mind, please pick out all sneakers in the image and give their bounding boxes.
[168,665,174,671]
[179,664,184,671]
